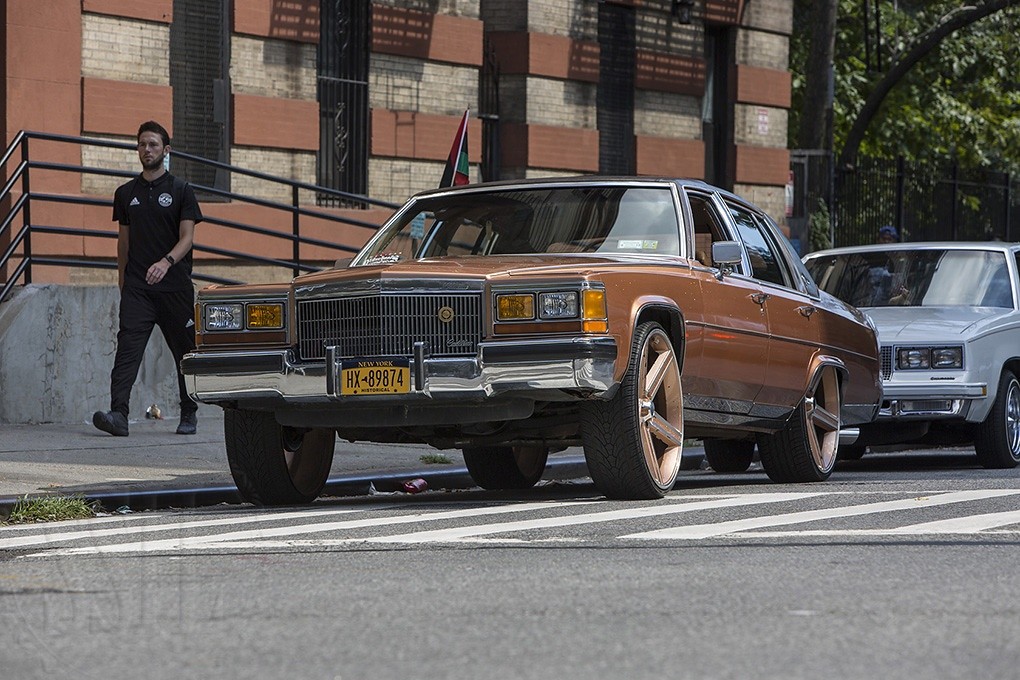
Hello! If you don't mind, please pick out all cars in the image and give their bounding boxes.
[182,174,886,500]
[790,243,1020,463]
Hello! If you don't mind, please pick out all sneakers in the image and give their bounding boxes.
[177,409,199,434]
[92,410,129,437]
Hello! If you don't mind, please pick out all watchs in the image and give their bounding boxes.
[164,255,175,266]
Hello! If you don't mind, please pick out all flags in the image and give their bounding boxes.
[438,111,469,188]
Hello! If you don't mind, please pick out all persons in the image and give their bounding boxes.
[92,121,203,437]
[860,225,900,297]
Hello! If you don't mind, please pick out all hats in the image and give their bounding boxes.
[879,225,898,239]
